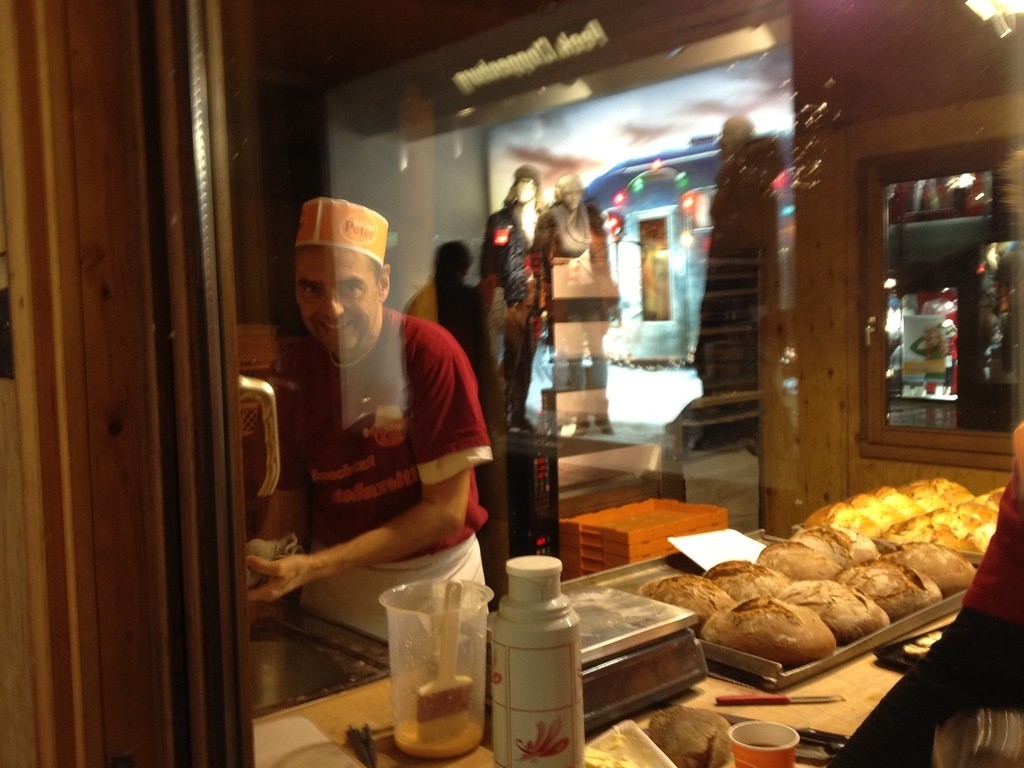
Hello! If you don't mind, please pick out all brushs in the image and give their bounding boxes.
[412,578,473,741]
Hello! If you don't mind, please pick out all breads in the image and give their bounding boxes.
[635,525,978,666]
[649,709,732,767]
[808,476,1008,561]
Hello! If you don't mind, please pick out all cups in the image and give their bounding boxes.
[380,578,498,760]
[728,719,800,768]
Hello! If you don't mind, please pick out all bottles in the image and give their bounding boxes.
[493,557,584,768]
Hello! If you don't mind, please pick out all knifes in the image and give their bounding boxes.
[716,695,845,705]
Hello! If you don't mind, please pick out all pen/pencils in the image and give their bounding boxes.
[345,722,378,768]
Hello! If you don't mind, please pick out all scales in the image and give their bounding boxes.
[485,579,712,745]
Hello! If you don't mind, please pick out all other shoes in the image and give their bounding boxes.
[510,418,537,433]
[593,399,613,435]
[570,413,591,438]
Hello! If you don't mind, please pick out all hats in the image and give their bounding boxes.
[515,165,540,183]
[295,197,388,268]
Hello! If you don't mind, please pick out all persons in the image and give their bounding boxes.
[245,197,494,701]
[401,237,503,448]
[531,176,621,437]
[693,114,785,449]
[482,166,549,434]
[831,421,1024,767]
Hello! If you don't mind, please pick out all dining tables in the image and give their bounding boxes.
[252,613,960,768]
